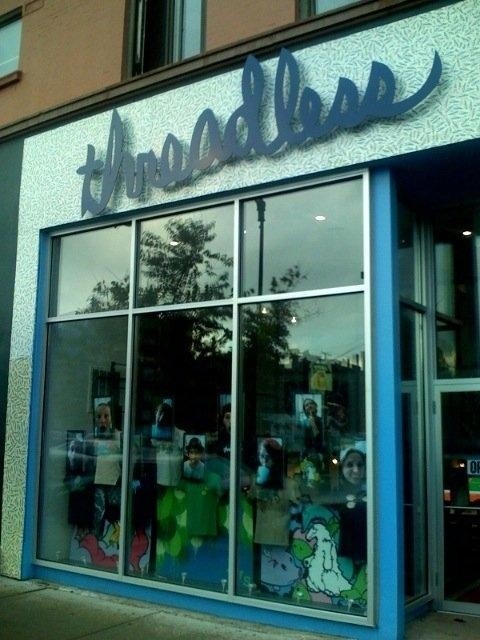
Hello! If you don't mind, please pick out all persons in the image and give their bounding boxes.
[66,398,366,498]
